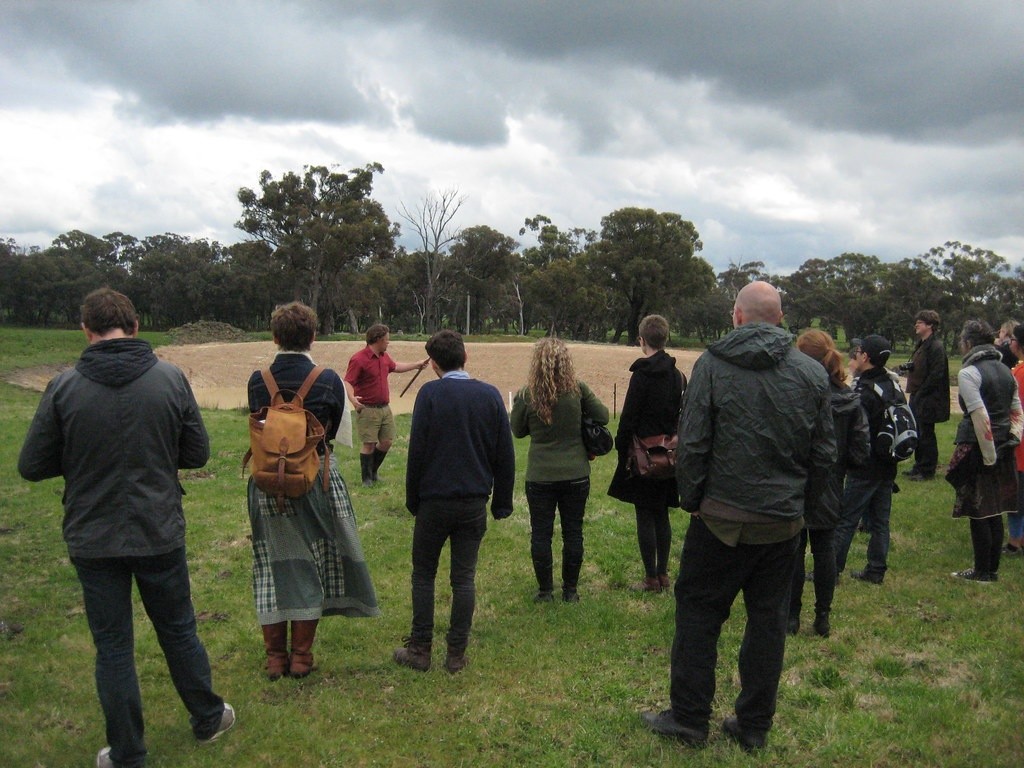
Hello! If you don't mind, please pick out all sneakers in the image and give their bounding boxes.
[641,709,708,746]
[950,567,998,585]
[195,703,235,745]
[804,570,839,585]
[96,746,115,768]
[722,716,766,753]
[851,570,884,584]
[1000,544,1024,559]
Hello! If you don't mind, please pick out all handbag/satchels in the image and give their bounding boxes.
[626,432,678,481]
[580,383,613,456]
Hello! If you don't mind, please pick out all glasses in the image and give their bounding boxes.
[854,348,864,355]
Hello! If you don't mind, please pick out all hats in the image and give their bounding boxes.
[852,334,892,368]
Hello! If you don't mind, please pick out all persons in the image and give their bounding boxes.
[247,301,382,680]
[344,324,430,488]
[511,336,609,603]
[994,319,1021,369]
[805,335,906,585]
[607,314,687,592]
[393,330,515,676]
[787,329,871,637]
[946,320,1024,584]
[902,311,951,480]
[1002,325,1024,556]
[654,281,839,752]
[18,288,236,768]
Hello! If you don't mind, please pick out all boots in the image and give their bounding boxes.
[373,447,385,482]
[290,619,319,678]
[394,636,433,672]
[262,620,290,681]
[447,644,470,672]
[360,453,375,488]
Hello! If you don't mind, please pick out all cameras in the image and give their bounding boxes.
[899,362,914,372]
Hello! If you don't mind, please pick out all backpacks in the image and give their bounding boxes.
[242,365,328,513]
[860,377,918,464]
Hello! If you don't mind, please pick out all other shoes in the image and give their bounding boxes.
[660,574,672,590]
[534,592,554,605]
[630,574,659,594]
[813,611,829,637]
[562,593,580,603]
[787,614,799,635]
[901,467,935,481]
